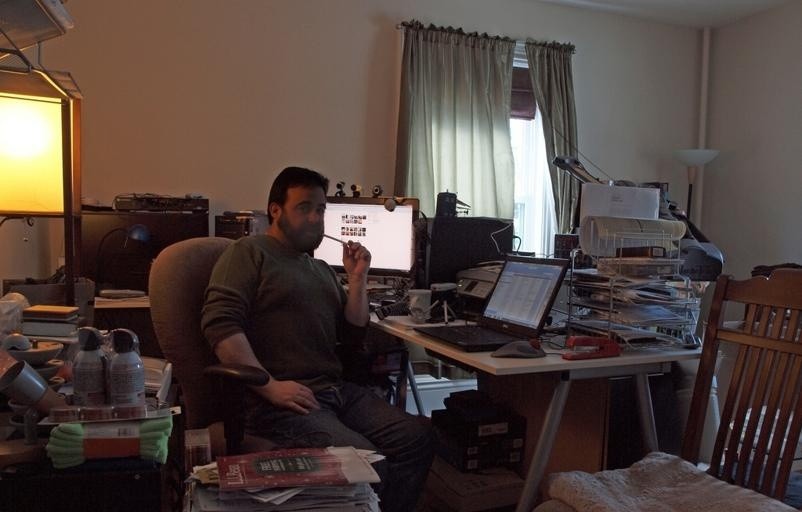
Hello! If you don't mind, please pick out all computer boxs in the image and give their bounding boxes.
[413,216,514,289]
[215,214,269,240]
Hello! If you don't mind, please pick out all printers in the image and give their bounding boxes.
[550,155,723,281]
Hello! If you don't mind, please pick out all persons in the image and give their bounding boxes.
[197,163,441,512]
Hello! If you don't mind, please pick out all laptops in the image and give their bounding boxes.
[414,257,570,352]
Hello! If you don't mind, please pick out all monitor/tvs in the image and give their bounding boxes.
[306,196,420,293]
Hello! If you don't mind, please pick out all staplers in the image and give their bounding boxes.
[563,335,621,359]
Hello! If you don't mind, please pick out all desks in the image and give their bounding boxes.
[342,299,711,509]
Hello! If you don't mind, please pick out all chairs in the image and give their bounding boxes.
[148,237,407,453]
[533,267,801,511]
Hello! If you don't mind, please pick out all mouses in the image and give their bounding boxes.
[491,338,546,358]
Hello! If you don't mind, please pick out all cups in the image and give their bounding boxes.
[405,288,434,323]
[1,361,67,416]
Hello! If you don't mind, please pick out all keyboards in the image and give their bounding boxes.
[375,301,411,321]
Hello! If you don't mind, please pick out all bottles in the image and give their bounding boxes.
[72,327,146,408]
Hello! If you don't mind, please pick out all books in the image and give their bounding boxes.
[188,446,387,512]
[562,183,696,330]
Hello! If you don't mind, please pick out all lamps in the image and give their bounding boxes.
[0,63,83,221]
[673,147,720,220]
